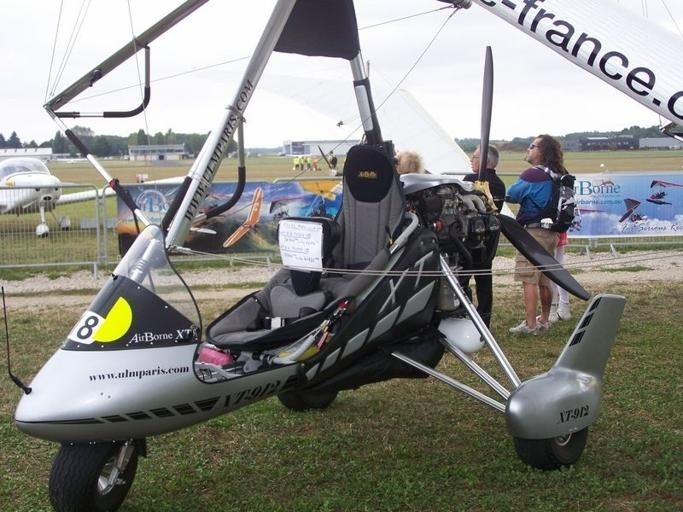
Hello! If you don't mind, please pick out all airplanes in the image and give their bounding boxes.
[0,153,186,239]
[6,1,679,512]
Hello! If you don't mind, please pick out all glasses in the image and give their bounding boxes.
[472,155,480,160]
[528,143,540,149]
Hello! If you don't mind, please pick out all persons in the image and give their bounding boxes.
[293,150,338,171]
[396,154,423,176]
[462,145,506,330]
[508,134,564,337]
[535,141,572,323]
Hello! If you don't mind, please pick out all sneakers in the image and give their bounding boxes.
[535,317,551,329]
[508,320,540,336]
[557,310,572,321]
[537,313,559,323]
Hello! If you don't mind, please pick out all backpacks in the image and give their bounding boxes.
[527,164,575,233]
[330,155,337,165]
